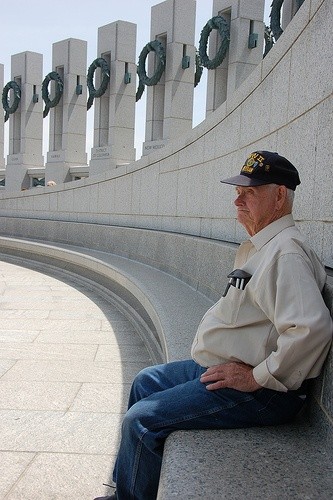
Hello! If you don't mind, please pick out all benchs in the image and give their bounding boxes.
[0,217,333,500]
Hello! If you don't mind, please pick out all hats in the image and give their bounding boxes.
[221,150,301,191]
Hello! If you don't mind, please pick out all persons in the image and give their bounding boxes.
[92,150,333,499]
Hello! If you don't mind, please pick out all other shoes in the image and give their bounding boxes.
[93,484,117,500]
